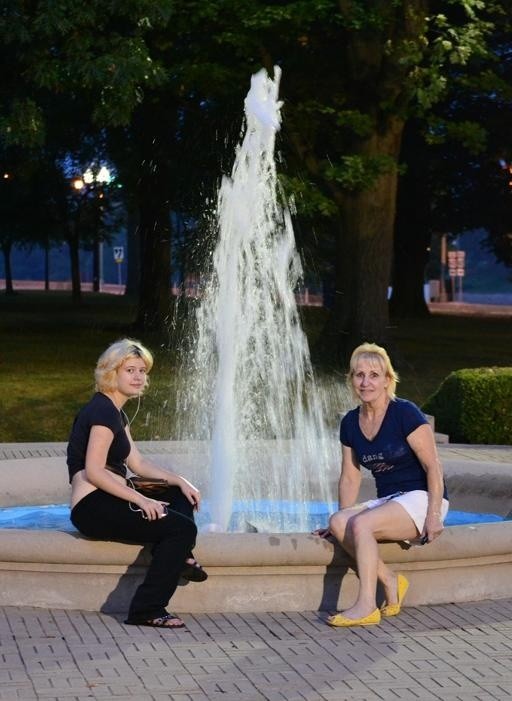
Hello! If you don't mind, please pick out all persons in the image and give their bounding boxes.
[65,336,210,629]
[307,342,448,629]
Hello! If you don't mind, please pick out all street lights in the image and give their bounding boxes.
[82,164,111,291]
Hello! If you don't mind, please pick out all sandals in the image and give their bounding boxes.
[378,573,409,616]
[325,607,382,628]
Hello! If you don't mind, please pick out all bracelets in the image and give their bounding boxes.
[427,511,442,516]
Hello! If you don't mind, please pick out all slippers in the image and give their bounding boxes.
[123,613,186,628]
[179,558,208,583]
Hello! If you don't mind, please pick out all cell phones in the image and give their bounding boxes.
[420,535,427,545]
[140,504,169,520]
